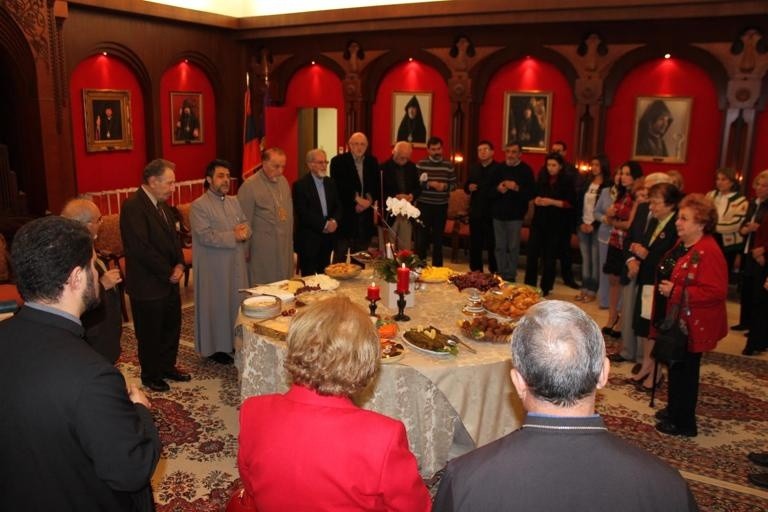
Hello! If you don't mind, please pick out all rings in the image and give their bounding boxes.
[636,252,639,254]
[660,292,663,294]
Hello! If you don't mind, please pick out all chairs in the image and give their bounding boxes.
[177,203,197,288]
[93,213,129,322]
[520,200,535,255]
[445,189,472,263]
[0,233,25,321]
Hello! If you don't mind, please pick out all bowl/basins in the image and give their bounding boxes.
[322,263,361,281]
[240,293,282,319]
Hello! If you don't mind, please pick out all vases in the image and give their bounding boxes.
[396,219,412,252]
[388,281,415,308]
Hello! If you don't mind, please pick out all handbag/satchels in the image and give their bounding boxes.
[649,287,690,369]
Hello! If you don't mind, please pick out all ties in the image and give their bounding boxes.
[157,201,167,223]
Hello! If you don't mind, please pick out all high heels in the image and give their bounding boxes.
[624,372,650,384]
[602,315,620,335]
[610,329,621,338]
[636,373,665,392]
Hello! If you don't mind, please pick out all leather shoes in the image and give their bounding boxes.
[161,368,192,381]
[142,375,171,391]
[210,352,235,365]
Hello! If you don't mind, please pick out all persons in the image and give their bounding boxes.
[730,171,768,356]
[463,141,501,274]
[550,142,581,289]
[227,295,432,512]
[706,168,750,268]
[291,149,342,277]
[434,300,699,512]
[638,100,674,157]
[0,216,164,512]
[331,133,380,263]
[177,98,198,139]
[95,103,120,139]
[491,144,537,282]
[238,146,294,286]
[747,449,768,488]
[61,198,122,364]
[414,138,457,267]
[192,160,253,365]
[118,158,190,393]
[377,142,422,252]
[524,149,584,298]
[578,153,729,437]
[397,95,426,142]
[510,99,543,146]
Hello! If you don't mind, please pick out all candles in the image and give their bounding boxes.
[396,263,410,294]
[367,281,379,300]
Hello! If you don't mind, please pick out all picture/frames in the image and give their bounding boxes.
[82,88,133,152]
[631,96,693,164]
[391,92,433,148]
[170,91,204,145]
[503,91,552,154]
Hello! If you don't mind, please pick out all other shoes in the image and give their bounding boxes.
[542,290,549,297]
[564,280,579,289]
[574,291,587,301]
[608,353,626,362]
[742,346,768,355]
[656,415,698,436]
[631,363,642,374]
[582,295,595,303]
[655,407,678,420]
[597,305,609,309]
[731,324,748,330]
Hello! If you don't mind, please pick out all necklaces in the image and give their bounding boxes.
[263,177,287,222]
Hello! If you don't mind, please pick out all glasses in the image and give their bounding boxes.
[677,216,698,221]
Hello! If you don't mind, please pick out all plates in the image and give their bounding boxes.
[376,338,406,363]
[417,276,448,284]
[480,303,511,321]
[303,274,341,291]
[400,328,450,355]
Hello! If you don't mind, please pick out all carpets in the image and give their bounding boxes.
[85,292,768,512]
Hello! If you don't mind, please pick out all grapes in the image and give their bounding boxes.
[462,317,513,337]
[448,270,501,292]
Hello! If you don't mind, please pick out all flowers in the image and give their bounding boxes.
[385,196,426,228]
[356,247,423,282]
